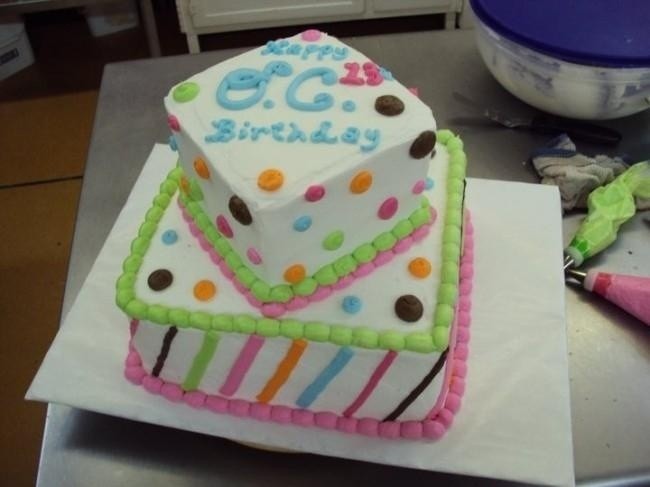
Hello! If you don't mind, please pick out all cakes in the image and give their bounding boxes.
[115,28,473,438]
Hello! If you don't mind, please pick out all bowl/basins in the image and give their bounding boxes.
[470,1,650,122]
